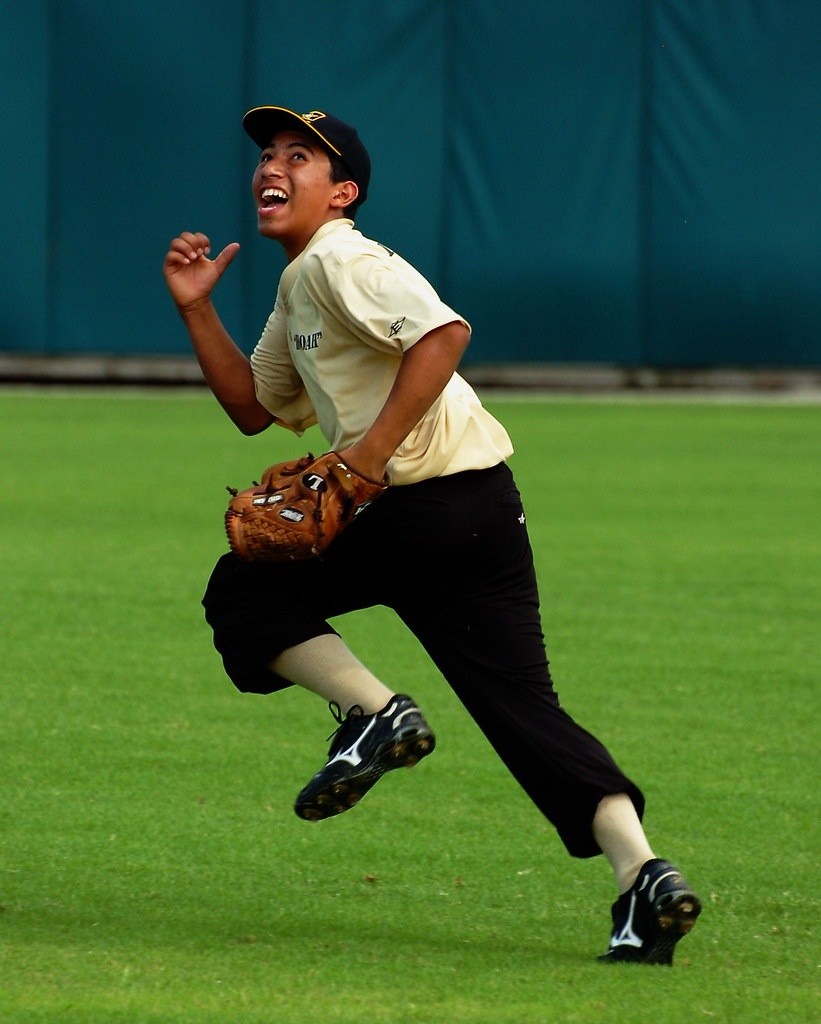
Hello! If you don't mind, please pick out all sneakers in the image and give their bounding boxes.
[599,858,702,967]
[294,693,435,821]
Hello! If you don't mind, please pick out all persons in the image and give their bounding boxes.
[161,105,700,962]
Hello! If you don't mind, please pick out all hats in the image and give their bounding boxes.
[242,106,371,202]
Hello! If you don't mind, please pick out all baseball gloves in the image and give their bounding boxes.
[225,447,392,562]
